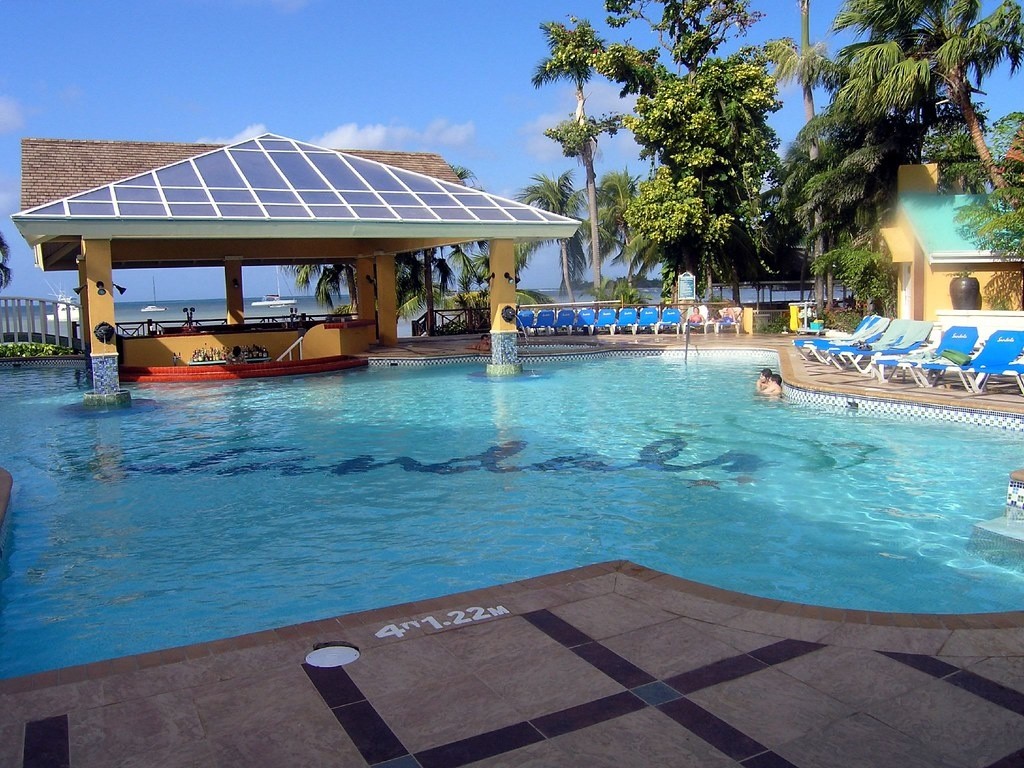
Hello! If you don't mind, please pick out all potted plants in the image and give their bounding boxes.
[947,269,980,309]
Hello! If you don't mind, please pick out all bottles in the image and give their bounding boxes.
[191,342,268,361]
[178,352,181,365]
[173,353,176,366]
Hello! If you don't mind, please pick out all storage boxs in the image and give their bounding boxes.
[810,322,824,330]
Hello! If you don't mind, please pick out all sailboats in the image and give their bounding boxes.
[141,276,168,312]
[251,266,297,307]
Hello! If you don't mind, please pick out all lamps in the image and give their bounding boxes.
[113,284,127,295]
[483,272,521,284]
[73,284,87,294]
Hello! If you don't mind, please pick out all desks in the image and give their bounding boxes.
[189,357,272,366]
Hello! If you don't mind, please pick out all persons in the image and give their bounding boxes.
[757,369,782,394]
[226,346,247,365]
[684,307,705,341]
[466,334,490,351]
[712,307,742,326]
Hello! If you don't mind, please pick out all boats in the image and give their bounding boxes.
[42,278,79,322]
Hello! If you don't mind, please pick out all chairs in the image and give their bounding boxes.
[791,315,1024,397]
[515,307,743,337]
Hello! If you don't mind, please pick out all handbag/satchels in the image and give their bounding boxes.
[934,349,971,366]
